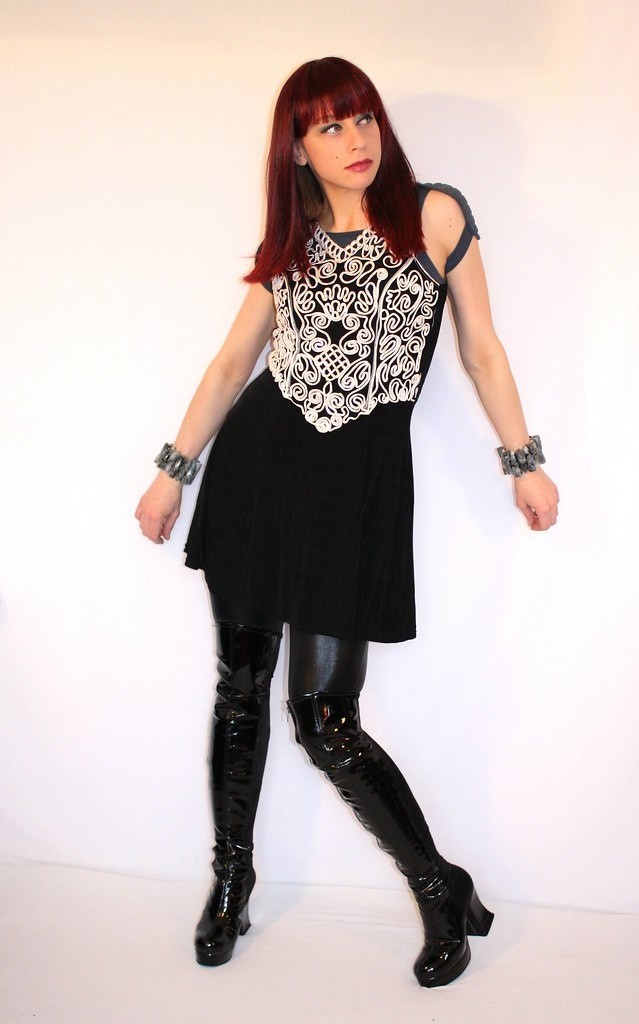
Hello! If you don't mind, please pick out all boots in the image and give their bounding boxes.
[287,692,494,987]
[194,622,283,966]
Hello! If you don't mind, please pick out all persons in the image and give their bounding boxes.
[136,57,559,988]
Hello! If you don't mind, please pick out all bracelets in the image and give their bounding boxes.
[154,443,202,485]
[496,435,547,477]
